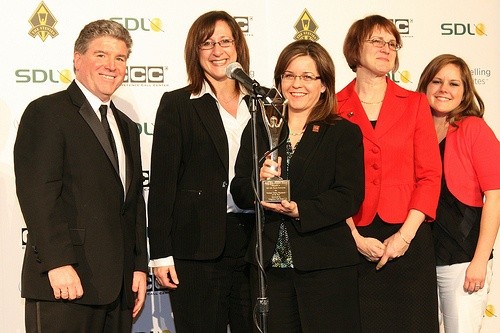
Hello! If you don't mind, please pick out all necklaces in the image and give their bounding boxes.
[289,130,304,136]
[361,100,384,105]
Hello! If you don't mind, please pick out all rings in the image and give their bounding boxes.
[475,285,480,287]
[61,290,67,294]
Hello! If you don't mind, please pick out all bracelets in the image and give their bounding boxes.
[399,230,410,244]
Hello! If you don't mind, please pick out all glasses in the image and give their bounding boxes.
[198,37,237,49]
[280,73,321,82]
[361,38,402,51]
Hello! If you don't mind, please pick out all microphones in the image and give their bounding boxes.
[225,62,266,96]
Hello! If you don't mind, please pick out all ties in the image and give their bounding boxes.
[98,104,119,176]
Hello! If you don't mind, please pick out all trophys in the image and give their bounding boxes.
[258,98,290,202]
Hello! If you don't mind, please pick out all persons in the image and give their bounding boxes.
[417,54,500,333]
[229,39,366,333]
[147,11,273,333]
[326,15,443,333]
[13,21,150,333]
